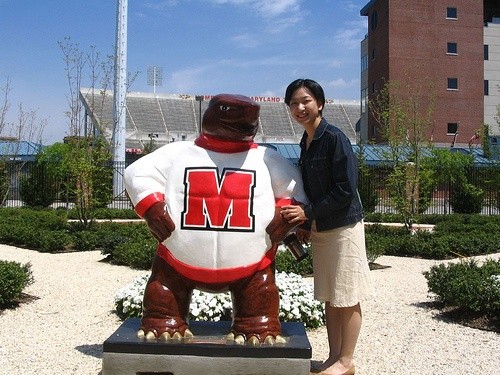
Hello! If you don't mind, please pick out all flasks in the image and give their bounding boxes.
[283,232,309,262]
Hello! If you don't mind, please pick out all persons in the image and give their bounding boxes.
[279,79,369,375]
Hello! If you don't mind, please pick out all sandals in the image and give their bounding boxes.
[311,362,355,375]
